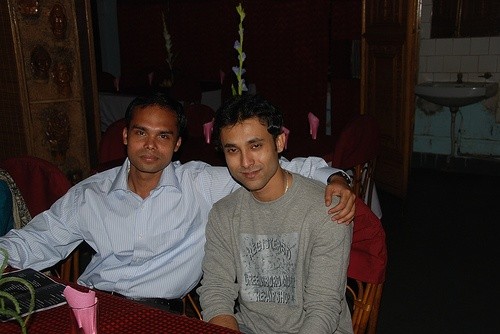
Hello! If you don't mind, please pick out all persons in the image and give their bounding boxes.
[0,94,356,315]
[195,93,356,333]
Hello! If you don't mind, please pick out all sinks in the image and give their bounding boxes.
[412,81,500,107]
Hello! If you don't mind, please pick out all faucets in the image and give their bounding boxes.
[457,72,463,83]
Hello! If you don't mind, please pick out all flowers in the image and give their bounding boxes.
[231,3,248,94]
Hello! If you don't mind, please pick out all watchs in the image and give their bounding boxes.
[326,171,351,189]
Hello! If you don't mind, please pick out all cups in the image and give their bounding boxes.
[67,297,100,334]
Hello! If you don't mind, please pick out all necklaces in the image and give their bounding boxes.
[281,171,290,194]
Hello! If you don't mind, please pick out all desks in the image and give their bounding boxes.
[0,267,237,334]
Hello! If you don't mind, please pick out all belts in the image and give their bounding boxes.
[101,290,183,313]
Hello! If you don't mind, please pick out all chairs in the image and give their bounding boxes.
[324,114,378,207]
[344,192,389,334]
[0,156,79,283]
